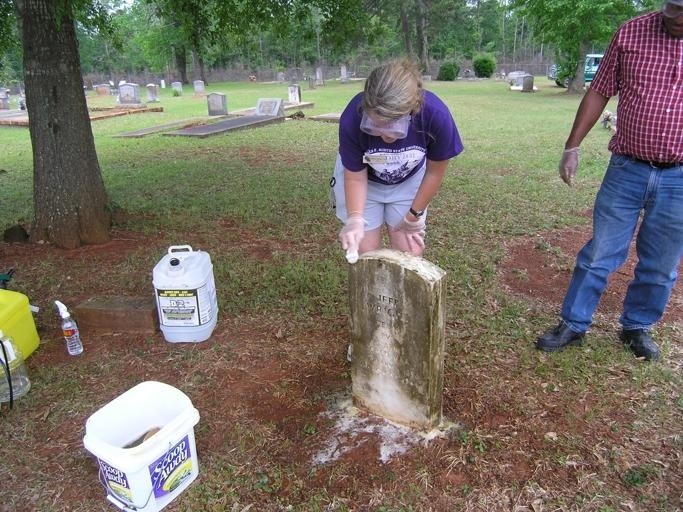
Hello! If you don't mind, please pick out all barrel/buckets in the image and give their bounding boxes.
[152,244,219,343]
[1,332,30,402]
[82,382,202,511]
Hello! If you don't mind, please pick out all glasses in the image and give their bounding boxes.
[359,108,411,140]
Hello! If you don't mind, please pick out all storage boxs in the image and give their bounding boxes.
[1,287,41,377]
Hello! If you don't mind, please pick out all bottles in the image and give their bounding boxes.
[61,311,83,355]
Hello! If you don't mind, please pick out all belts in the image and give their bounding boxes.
[623,153,683,170]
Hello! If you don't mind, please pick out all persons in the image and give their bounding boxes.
[532,1,683,364]
[329,52,465,364]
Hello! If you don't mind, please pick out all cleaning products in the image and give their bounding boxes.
[53,299,83,355]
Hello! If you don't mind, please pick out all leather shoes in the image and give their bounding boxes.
[536,321,586,353]
[621,325,659,361]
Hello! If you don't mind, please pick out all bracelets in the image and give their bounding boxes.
[408,207,424,219]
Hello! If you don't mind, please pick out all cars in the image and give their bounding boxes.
[547,53,604,88]
